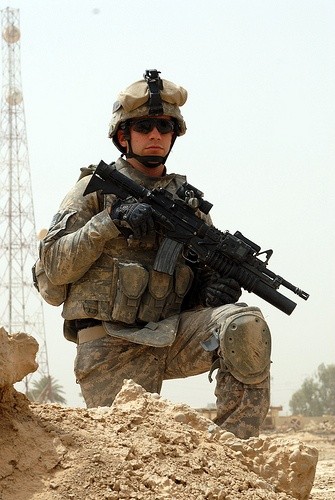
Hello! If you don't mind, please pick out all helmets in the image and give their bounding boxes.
[108,79,187,138]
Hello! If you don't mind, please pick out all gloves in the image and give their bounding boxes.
[206,278,242,308]
[111,203,174,240]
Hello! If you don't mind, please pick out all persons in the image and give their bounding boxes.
[31,68,272,439]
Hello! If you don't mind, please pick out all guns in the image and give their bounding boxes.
[82,159,310,316]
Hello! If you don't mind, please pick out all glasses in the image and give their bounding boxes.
[128,118,175,134]
